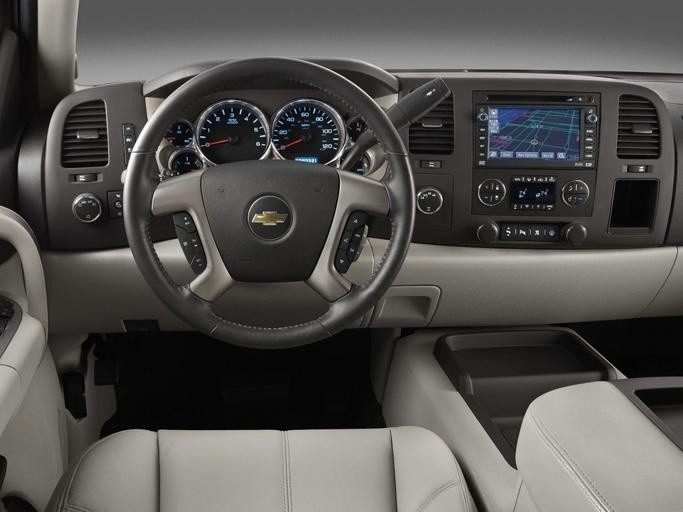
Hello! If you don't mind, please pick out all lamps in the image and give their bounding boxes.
[50,425,477,512]
